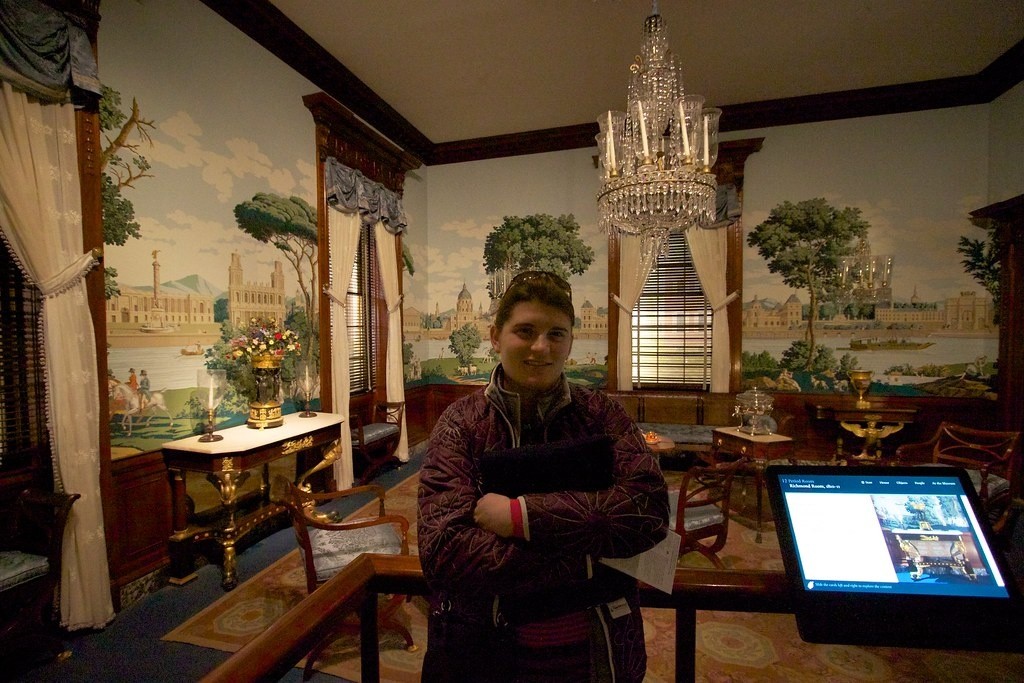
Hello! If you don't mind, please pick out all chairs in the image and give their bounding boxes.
[0,487,80,654]
[894,422,1022,553]
[664,459,750,572]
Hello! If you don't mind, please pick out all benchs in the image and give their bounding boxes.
[601,391,746,427]
[349,393,406,486]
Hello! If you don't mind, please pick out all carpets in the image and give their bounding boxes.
[162,471,1024,683]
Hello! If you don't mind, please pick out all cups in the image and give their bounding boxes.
[646,431,658,444]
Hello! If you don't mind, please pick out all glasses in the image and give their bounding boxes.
[502,270,572,302]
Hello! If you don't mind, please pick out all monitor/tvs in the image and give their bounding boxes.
[765,463,1024,654]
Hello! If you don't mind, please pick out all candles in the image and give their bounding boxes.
[208,378,213,409]
[305,365,309,392]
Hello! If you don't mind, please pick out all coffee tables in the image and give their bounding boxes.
[630,423,738,465]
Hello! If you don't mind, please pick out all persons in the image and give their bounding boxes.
[417,270,670,683]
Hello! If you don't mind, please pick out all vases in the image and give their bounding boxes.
[245,352,285,428]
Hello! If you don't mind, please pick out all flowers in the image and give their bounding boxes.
[225,315,303,367]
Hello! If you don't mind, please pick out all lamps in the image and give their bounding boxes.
[838,244,895,303]
[591,0,723,276]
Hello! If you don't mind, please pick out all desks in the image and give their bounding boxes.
[707,424,796,543]
[645,434,674,463]
[804,399,917,464]
[156,406,344,591]
